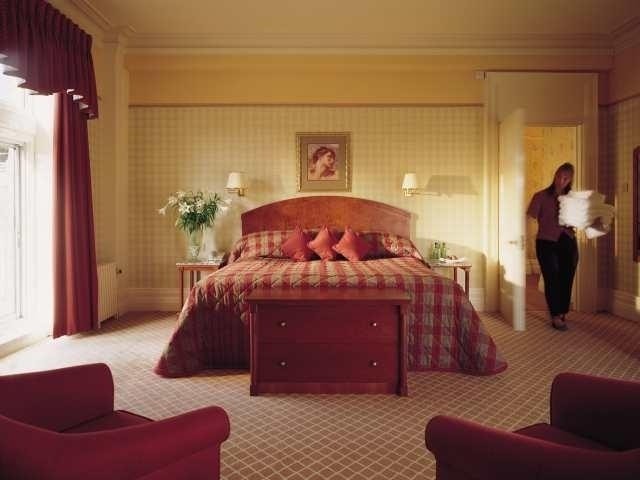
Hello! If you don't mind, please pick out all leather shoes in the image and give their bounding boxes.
[551,314,569,331]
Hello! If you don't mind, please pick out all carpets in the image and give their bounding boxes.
[0,308,639,480]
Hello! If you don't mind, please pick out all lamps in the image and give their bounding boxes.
[402,174,418,197]
[226,172,248,196]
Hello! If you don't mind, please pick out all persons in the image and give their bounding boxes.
[526,162,602,332]
[307,146,337,180]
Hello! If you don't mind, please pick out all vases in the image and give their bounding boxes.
[185,226,202,263]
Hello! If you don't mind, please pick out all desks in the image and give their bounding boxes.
[425,257,471,301]
[177,260,226,321]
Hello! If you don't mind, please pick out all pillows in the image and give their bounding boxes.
[332,225,372,261]
[281,225,314,261]
[307,224,340,260]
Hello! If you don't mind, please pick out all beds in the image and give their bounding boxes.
[153,195,508,378]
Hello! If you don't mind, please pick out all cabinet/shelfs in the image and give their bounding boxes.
[242,288,411,397]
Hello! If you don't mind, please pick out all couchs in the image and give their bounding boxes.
[0,363,229,480]
[426,373,640,479]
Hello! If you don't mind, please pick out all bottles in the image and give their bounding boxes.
[434,241,441,259]
[441,241,447,259]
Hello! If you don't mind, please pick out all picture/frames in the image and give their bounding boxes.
[295,132,352,192]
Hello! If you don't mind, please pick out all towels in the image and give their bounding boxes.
[558,190,614,238]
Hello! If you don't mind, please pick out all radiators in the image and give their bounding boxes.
[97,263,118,328]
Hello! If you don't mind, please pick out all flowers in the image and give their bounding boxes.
[158,190,230,231]
